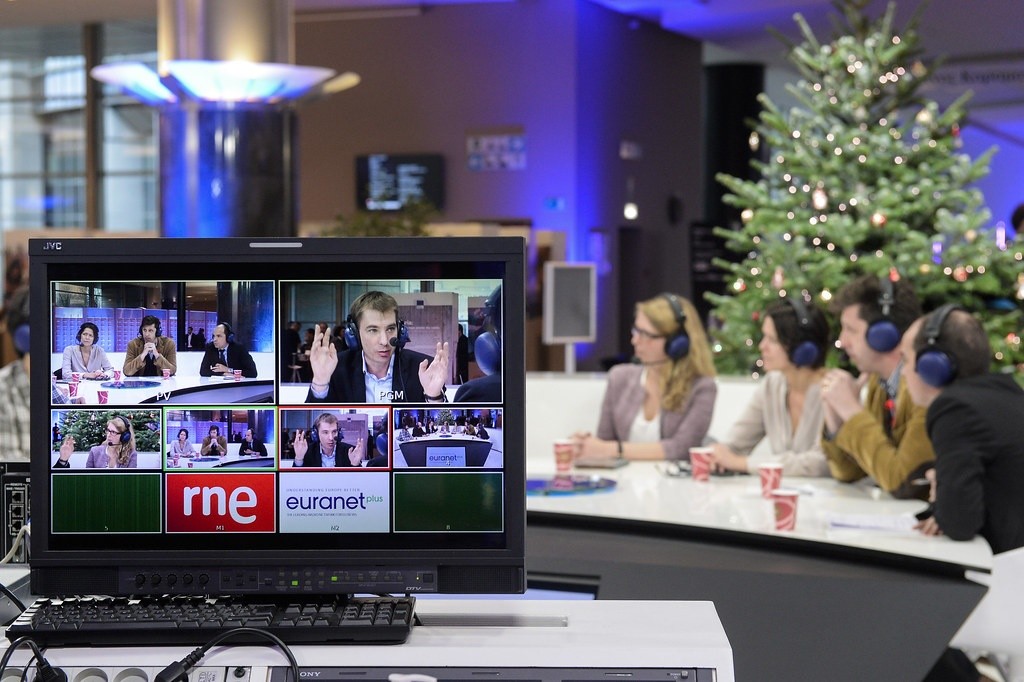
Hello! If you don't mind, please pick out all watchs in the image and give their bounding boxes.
[422,389,445,400]
[228,368,232,373]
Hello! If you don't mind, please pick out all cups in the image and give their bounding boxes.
[770,489,798,532]
[688,447,713,482]
[175,453,180,459]
[163,369,170,380]
[551,439,575,475]
[174,458,179,466]
[96,390,108,405]
[256,452,260,457]
[113,370,121,380]
[71,372,80,384]
[220,452,224,457]
[195,453,200,458]
[52,376,58,382]
[756,463,782,499]
[188,462,193,467]
[68,382,79,397]
[233,369,242,382]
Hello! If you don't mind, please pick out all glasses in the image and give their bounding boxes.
[631,324,666,340]
[104,429,122,437]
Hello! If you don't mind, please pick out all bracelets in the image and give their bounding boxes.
[312,382,329,386]
[614,438,622,460]
[59,458,67,462]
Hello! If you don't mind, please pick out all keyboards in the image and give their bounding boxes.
[6,597,416,650]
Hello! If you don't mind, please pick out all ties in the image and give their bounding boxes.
[219,349,227,367]
[249,442,251,450]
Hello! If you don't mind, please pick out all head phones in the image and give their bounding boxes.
[116,415,131,444]
[915,303,965,388]
[76,323,99,344]
[209,430,221,436]
[310,427,344,443]
[786,297,819,366]
[251,427,257,439]
[660,290,690,359]
[344,315,409,352]
[866,278,901,352]
[376,414,388,456]
[475,285,501,376]
[220,321,234,343]
[139,320,162,337]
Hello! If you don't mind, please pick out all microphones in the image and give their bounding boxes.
[137,332,144,341]
[334,436,344,442]
[108,440,127,446]
[76,339,84,347]
[390,337,411,346]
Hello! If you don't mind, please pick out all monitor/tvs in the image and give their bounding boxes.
[29,237,528,602]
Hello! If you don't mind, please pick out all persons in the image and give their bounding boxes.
[571,294,717,462]
[821,275,936,499]
[1,286,30,460]
[707,299,833,478]
[52,291,503,467]
[899,306,1023,555]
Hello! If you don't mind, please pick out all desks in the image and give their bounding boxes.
[526,459,993,681]
[399,433,493,467]
[178,455,275,468]
[76,375,274,405]
